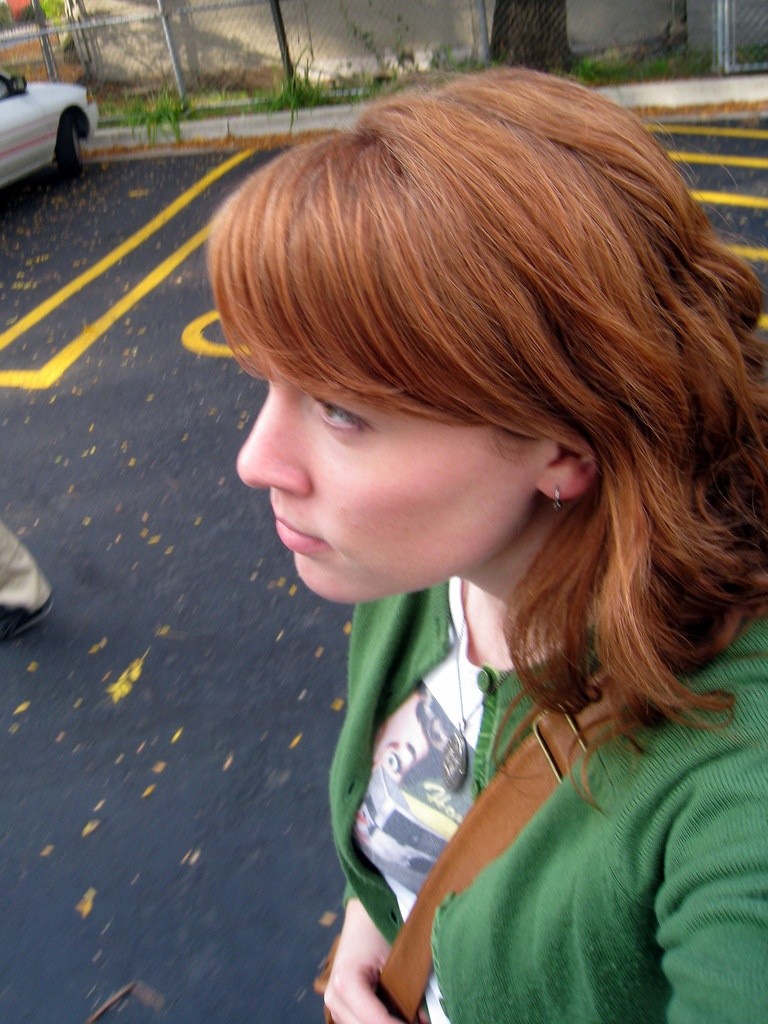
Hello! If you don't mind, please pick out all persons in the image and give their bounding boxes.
[0,522,54,642]
[207,68,768,1024]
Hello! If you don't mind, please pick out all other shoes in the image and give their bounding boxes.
[1,592,53,643]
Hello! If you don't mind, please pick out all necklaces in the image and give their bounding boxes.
[442,580,514,794]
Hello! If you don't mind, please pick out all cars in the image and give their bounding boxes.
[0,70,100,191]
